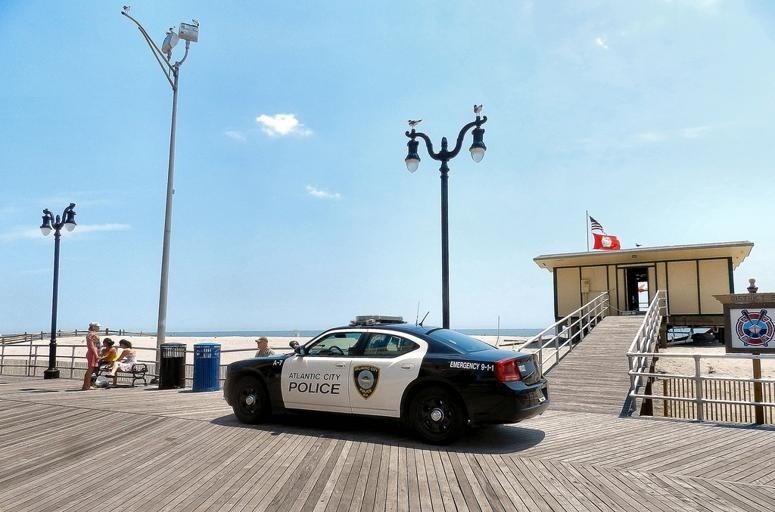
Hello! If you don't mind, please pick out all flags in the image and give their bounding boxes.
[592,232,621,251]
[589,217,606,234]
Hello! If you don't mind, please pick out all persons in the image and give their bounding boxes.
[82,322,102,390]
[105,339,137,387]
[90,338,118,384]
[254,337,274,357]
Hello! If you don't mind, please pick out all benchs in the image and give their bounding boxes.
[83,362,148,386]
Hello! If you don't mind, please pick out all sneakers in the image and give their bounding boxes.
[107,385,117,388]
[106,373,115,378]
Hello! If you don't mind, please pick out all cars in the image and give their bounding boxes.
[223,312,551,445]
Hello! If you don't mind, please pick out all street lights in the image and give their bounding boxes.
[40,202,77,379]
[405,103,487,329]
[121,4,200,380]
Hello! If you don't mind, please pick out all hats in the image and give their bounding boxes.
[88,322,102,327]
[255,337,268,343]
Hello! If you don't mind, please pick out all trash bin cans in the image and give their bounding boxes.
[193,342,221,392]
[158,342,187,390]
